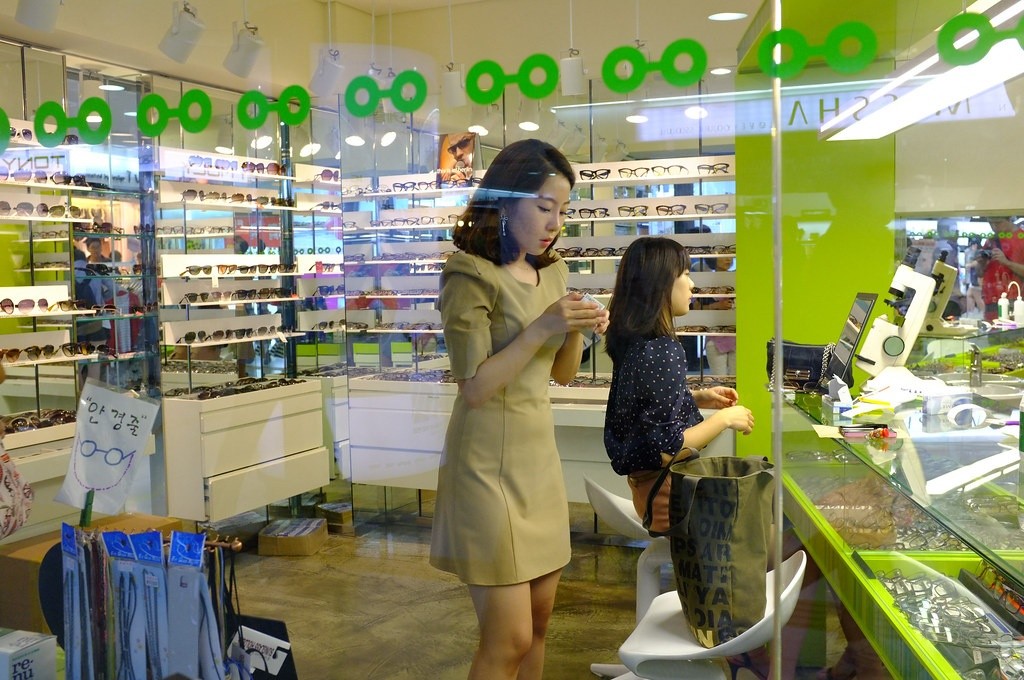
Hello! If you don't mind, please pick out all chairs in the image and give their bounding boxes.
[618,549,807,680]
[580,472,654,542]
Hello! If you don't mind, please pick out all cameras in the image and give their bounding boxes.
[981,251,993,259]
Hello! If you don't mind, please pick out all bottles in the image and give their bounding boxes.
[997,292,1010,320]
[1014,297,1024,328]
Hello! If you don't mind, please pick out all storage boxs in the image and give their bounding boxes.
[257,518,328,556]
[267,491,328,519]
[0,627,58,680]
[0,509,220,636]
[316,497,356,534]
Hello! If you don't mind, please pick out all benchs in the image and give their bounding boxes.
[634,535,674,625]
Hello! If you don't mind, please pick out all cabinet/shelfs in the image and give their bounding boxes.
[0,118,736,524]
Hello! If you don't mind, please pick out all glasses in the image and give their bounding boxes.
[565,209,576,219]
[694,203,729,215]
[369,219,393,227]
[578,207,611,219]
[783,444,1024,680]
[656,204,686,216]
[684,243,737,255]
[579,169,612,179]
[673,324,737,333]
[420,216,446,224]
[780,376,821,395]
[617,205,650,217]
[683,376,739,392]
[554,245,628,257]
[0,126,482,435]
[562,285,616,295]
[697,163,730,175]
[651,165,689,176]
[392,217,420,226]
[549,374,612,388]
[617,167,650,179]
[691,285,735,295]
[447,213,459,223]
[907,338,1024,378]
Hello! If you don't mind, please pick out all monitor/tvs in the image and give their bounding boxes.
[819,291,878,395]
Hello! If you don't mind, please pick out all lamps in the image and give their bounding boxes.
[291,125,312,157]
[344,109,365,147]
[364,0,380,78]
[308,0,346,101]
[368,114,395,146]
[248,123,273,151]
[222,0,264,80]
[381,0,402,115]
[517,86,541,132]
[625,85,649,124]
[158,0,206,64]
[558,0,587,96]
[80,64,107,123]
[15,0,64,33]
[440,0,469,110]
[682,74,709,121]
[623,0,652,89]
[215,122,235,154]
[468,99,491,136]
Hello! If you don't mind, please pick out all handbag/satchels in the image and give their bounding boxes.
[763,337,854,399]
[641,438,779,650]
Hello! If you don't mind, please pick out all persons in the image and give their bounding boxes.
[816,582,891,680]
[603,236,818,680]
[428,139,610,680]
[440,133,476,187]
[73,238,146,393]
[692,253,736,375]
[976,217,1024,322]
